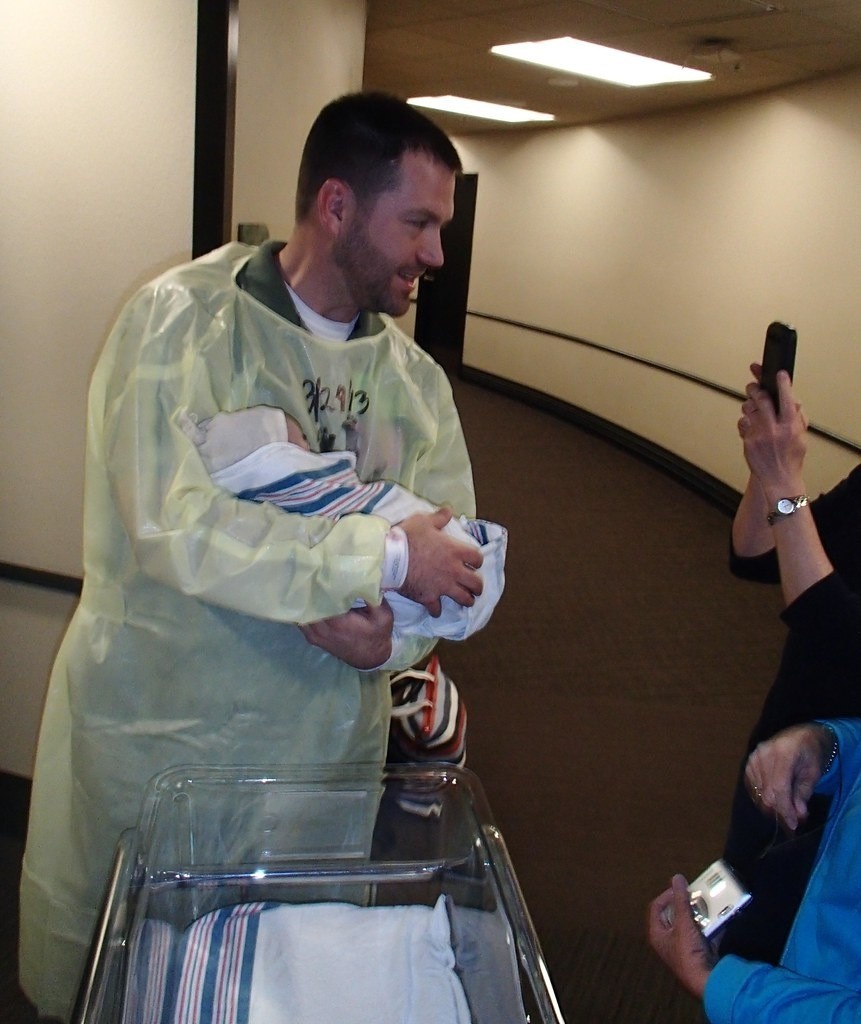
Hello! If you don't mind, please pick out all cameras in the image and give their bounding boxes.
[661,859,757,941]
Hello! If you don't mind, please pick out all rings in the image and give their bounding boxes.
[752,787,762,800]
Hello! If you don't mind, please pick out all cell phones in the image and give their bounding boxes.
[758,321,798,414]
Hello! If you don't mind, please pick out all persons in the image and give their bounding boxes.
[650,723,861,1024]
[195,405,508,642]
[17,91,476,1024]
[703,363,861,967]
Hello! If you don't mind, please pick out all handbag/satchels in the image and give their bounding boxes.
[389,656,468,770]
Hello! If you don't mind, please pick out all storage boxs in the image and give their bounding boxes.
[91,757,559,1024]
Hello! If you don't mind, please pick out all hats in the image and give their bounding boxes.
[178,405,289,476]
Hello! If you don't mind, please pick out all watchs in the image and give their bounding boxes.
[767,495,808,525]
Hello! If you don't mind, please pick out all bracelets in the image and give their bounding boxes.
[822,727,838,775]
